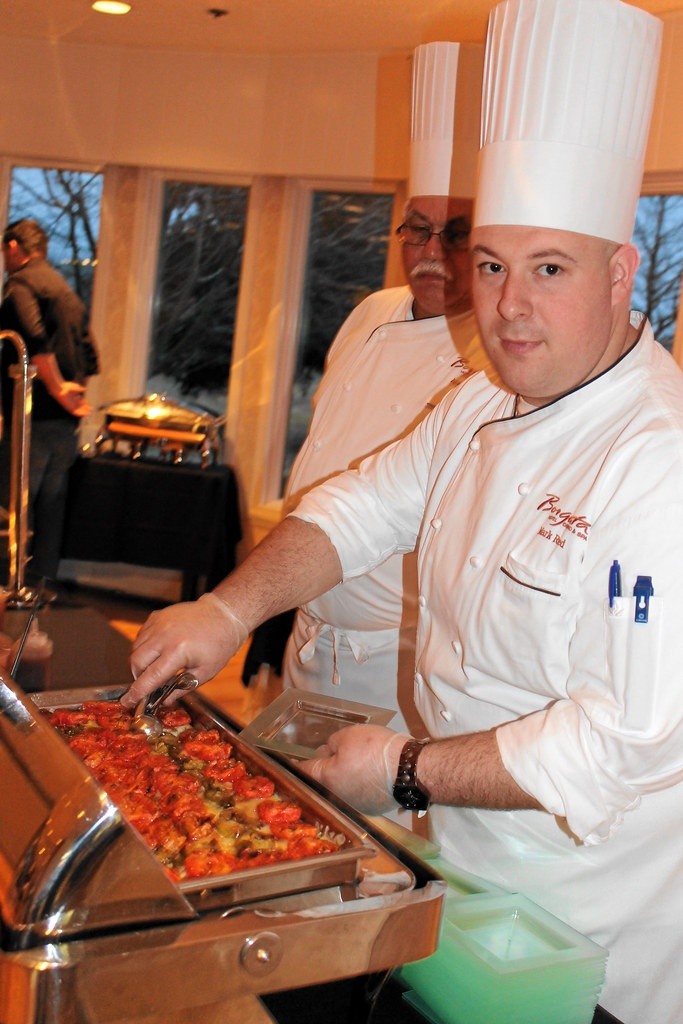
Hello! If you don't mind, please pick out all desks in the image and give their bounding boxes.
[0,607,625,1024]
[60,456,238,603]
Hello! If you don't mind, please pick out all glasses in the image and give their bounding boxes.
[395,223,471,249]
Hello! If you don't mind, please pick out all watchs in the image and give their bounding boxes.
[393,738,429,811]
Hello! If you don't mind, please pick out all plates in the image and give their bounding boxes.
[354,809,611,1024]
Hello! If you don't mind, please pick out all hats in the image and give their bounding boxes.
[470,0,665,244]
[407,41,487,199]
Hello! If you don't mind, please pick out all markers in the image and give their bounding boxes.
[633,576,654,623]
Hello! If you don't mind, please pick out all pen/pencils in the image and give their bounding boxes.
[606,559,621,608]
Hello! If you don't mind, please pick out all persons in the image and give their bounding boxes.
[0,218,100,610]
[281,183,490,832]
[116,161,683,1024]
